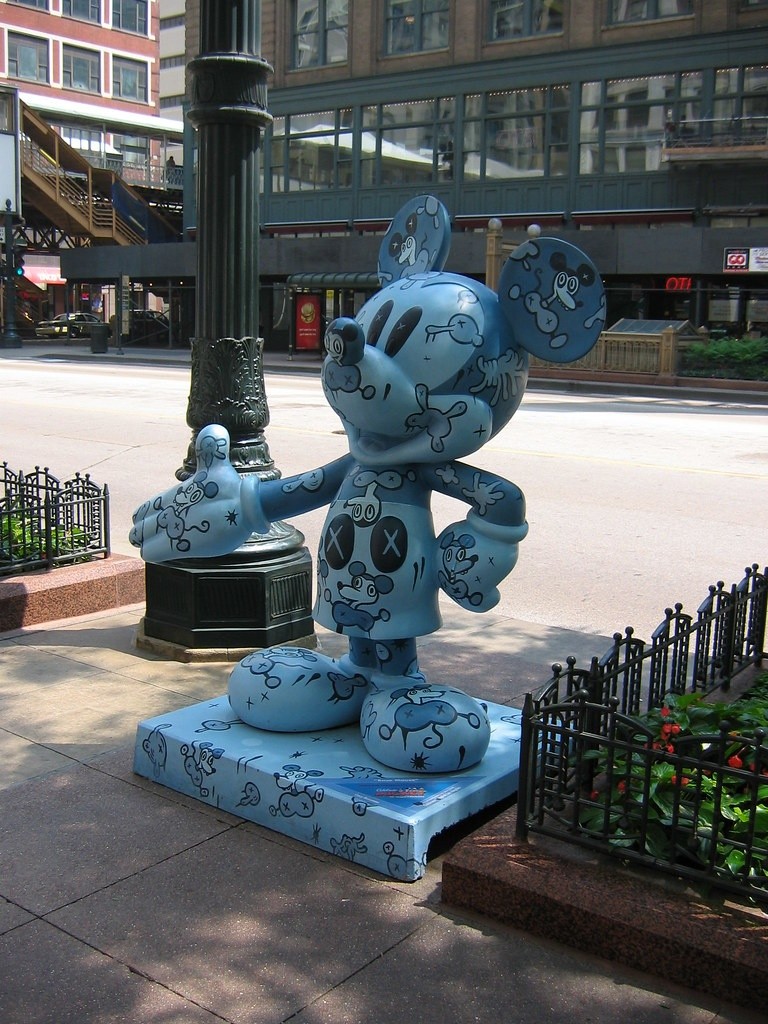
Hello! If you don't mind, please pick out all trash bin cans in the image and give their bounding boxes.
[90,322,109,354]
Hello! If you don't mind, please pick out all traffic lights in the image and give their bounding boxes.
[13,244,26,276]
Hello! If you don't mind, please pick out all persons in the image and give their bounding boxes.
[661,307,671,320]
[166,156,175,183]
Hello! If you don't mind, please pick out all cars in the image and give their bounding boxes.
[35,313,110,338]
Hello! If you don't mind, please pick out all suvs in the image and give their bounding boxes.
[107,309,169,340]
